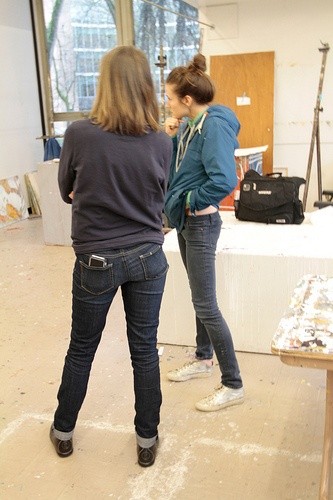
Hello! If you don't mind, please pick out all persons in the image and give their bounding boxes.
[164,54,244,413]
[48,45,173,466]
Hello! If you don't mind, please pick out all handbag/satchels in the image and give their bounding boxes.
[233,169,306,225]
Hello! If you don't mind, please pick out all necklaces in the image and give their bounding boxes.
[175,126,194,172]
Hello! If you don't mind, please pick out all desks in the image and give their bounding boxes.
[156,210,333,355]
[35,158,74,245]
[270,272,333,499]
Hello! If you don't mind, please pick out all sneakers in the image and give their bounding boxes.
[195,382,245,412]
[166,359,214,381]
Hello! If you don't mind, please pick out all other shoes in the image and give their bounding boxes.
[137,437,159,467]
[49,421,74,457]
